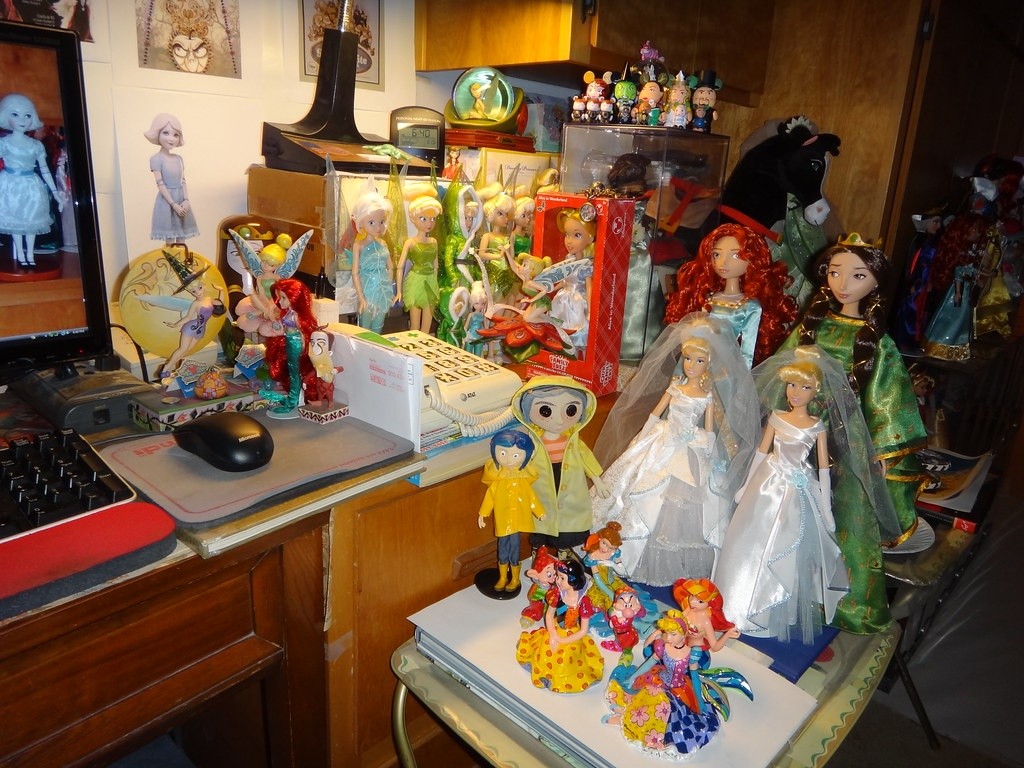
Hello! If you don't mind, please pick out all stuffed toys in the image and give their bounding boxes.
[722,115,841,230]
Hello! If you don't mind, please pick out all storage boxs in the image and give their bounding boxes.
[248,102,731,395]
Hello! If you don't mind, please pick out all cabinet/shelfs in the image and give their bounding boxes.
[634,0,1024,329]
[414,0,774,109]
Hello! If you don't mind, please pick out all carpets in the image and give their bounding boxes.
[390,699,1008,768]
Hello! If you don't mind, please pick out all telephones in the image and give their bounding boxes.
[327,320,524,435]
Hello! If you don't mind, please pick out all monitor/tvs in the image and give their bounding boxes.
[0,19,113,385]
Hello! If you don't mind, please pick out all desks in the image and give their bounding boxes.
[0,311,669,768]
[391,618,940,768]
[876,490,998,693]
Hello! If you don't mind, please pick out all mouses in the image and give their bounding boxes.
[173,412,274,471]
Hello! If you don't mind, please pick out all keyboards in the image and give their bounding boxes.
[0,426,137,542]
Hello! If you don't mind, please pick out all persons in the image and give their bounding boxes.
[570,79,718,132]
[590,312,772,587]
[160,250,224,378]
[895,161,1024,424]
[217,214,274,346]
[661,223,797,368]
[392,184,443,334]
[143,114,201,248]
[517,522,741,760]
[511,376,613,561]
[607,153,675,358]
[0,95,64,269]
[773,232,928,631]
[352,192,393,336]
[440,145,597,361]
[270,279,312,352]
[235,233,292,344]
[713,345,851,645]
[478,429,547,591]
